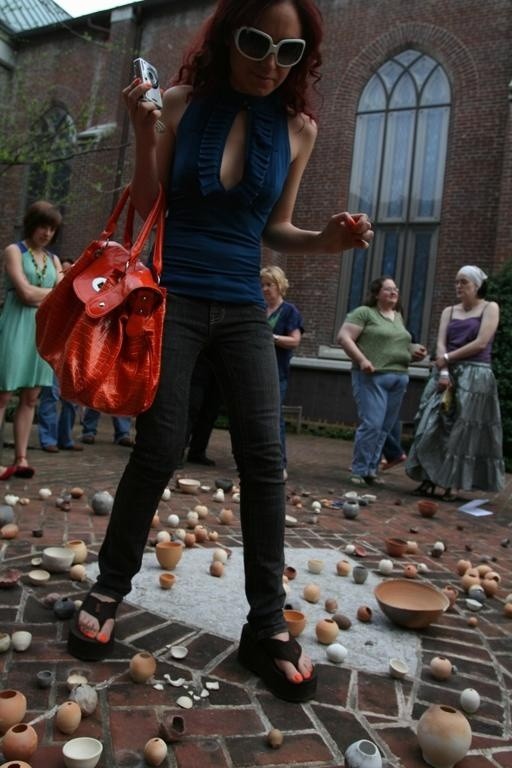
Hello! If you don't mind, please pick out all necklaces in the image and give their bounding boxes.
[21,241,50,288]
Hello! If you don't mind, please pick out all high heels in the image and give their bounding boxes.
[411,480,436,496]
[443,487,460,500]
[0,466,16,479]
[13,456,35,478]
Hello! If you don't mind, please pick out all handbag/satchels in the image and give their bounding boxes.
[36,178,166,417]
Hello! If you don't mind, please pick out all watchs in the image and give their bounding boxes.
[443,352,450,361]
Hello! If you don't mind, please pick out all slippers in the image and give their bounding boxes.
[365,476,385,485]
[347,474,368,487]
[67,580,119,660]
[241,625,316,703]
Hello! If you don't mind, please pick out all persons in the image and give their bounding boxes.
[81,406,136,446]
[69,1,374,702]
[380,416,407,470]
[259,266,304,481]
[406,266,506,503]
[39,258,83,453]
[338,276,428,490]
[0,201,65,480]
[186,383,221,467]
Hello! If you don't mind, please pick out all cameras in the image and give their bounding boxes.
[132,58,163,110]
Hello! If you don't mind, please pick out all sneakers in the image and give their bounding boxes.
[119,436,136,446]
[46,444,59,452]
[83,434,96,444]
[67,443,84,451]
[381,453,407,469]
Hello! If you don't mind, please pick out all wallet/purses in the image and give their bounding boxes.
[441,371,455,411]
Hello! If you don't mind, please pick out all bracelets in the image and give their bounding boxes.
[271,334,280,345]
[439,370,450,376]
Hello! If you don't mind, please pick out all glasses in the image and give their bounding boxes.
[262,282,277,288]
[232,27,307,67]
[381,287,399,292]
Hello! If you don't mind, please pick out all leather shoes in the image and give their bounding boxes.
[189,454,216,466]
